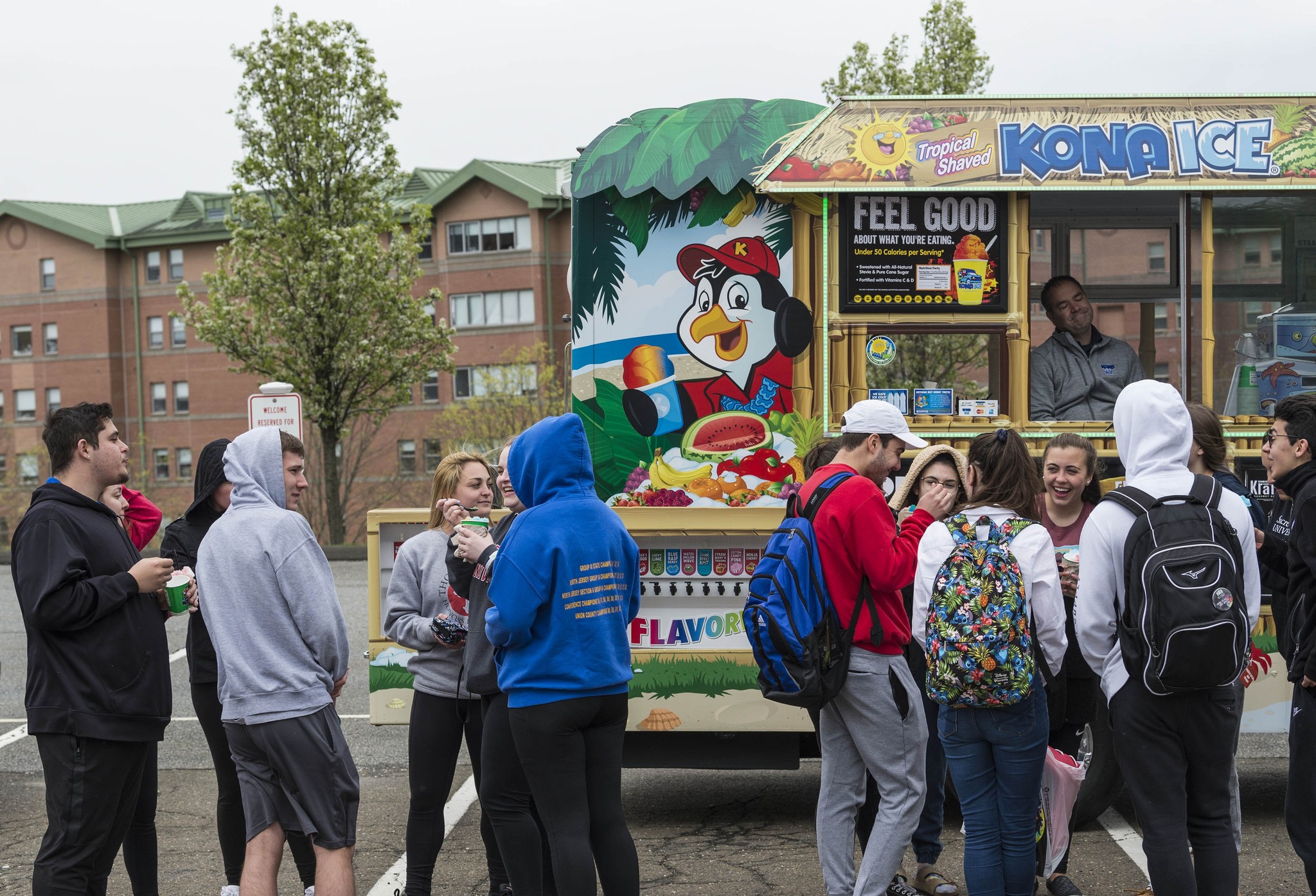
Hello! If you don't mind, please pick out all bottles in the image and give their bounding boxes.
[888,391,906,413]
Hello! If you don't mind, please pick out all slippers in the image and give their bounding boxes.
[911,866,959,896]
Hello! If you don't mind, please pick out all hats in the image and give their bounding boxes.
[841,398,929,450]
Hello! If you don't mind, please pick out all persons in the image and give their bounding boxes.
[11,399,199,896]
[1180,399,1266,575]
[1032,432,1103,896]
[97,480,185,895]
[1068,378,1262,895]
[801,436,922,895]
[159,438,318,895]
[1253,391,1315,896]
[435,434,552,896]
[482,412,641,896]
[383,452,514,896]
[1029,275,1149,420]
[194,425,360,895]
[912,428,1069,896]
[885,444,975,893]
[742,398,953,895]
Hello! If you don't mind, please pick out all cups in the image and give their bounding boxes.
[1062,554,1080,566]
[163,575,190,616]
[1236,363,1260,417]
[952,259,988,305]
[461,520,489,531]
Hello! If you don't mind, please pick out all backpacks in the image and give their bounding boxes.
[742,470,885,710]
[924,513,1041,708]
[1098,472,1254,696]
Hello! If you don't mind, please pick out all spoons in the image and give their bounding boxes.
[455,504,479,511]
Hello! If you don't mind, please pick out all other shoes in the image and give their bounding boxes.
[1045,875,1082,896]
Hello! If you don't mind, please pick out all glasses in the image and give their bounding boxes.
[920,477,961,495]
[1268,434,1311,455]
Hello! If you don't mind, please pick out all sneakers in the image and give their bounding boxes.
[888,876,920,895]
[1123,886,1155,896]
